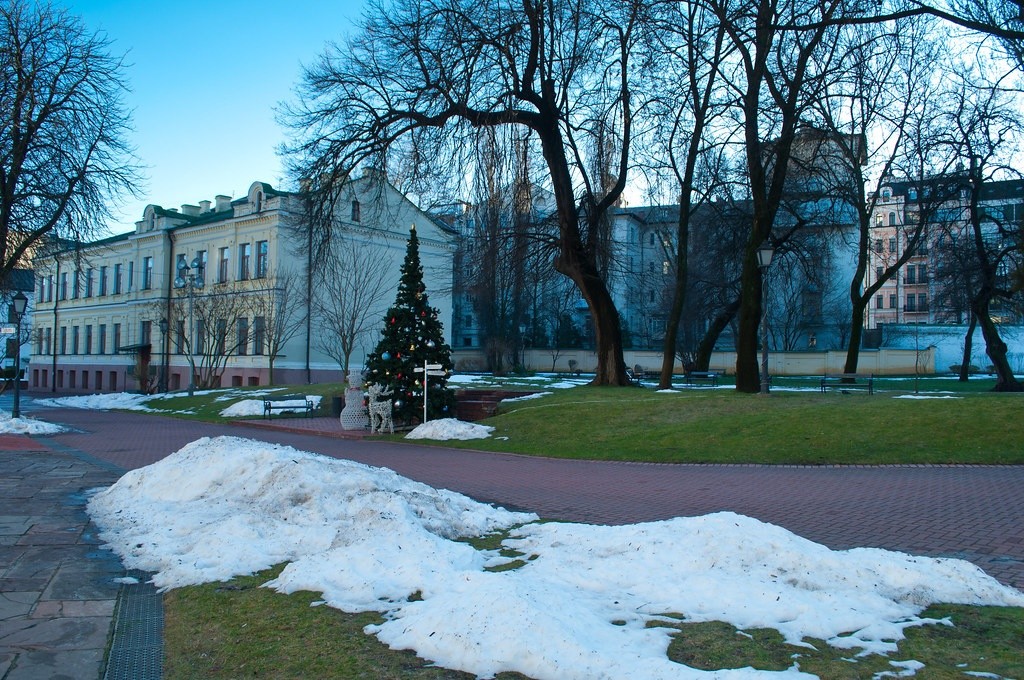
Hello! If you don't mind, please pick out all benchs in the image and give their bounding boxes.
[710,368,727,376]
[626,368,645,383]
[687,371,719,387]
[262,395,313,420]
[821,371,874,396]
